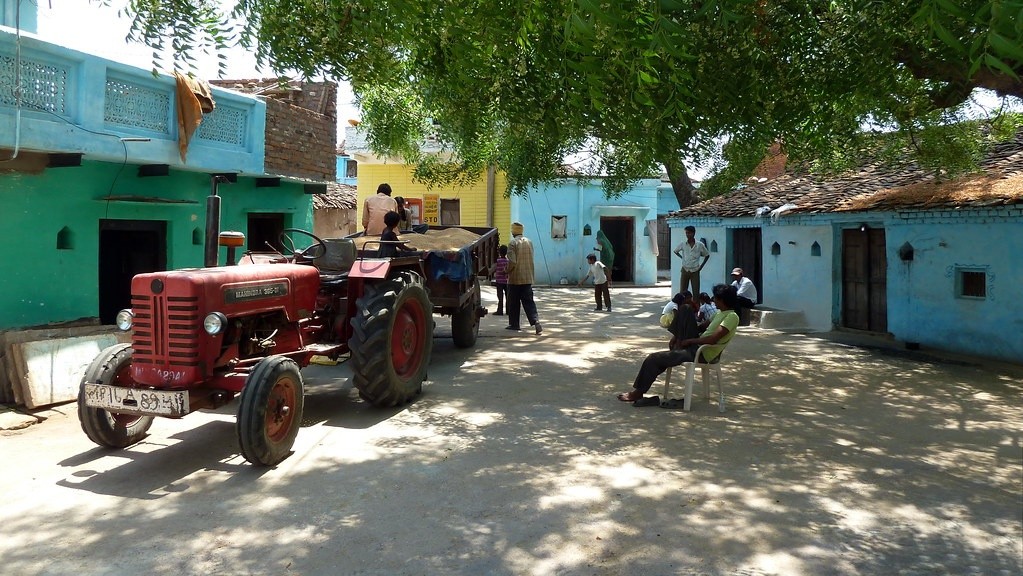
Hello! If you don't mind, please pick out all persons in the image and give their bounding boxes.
[490,245,509,316]
[394,195,429,235]
[502,221,543,334]
[617,283,740,401]
[376,211,417,259]
[660,291,720,328]
[673,225,710,301]
[576,229,615,314]
[728,268,757,317]
[361,183,398,234]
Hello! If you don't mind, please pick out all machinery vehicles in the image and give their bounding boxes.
[76,223,503,469]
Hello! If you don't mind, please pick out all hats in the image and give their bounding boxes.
[730,267,744,276]
[511,223,523,234]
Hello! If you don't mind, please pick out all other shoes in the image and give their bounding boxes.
[505,326,520,330]
[607,308,611,312]
[594,307,602,311]
[660,398,684,409]
[492,312,503,315]
[632,396,659,406]
[535,324,543,335]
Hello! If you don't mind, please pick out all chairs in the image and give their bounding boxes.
[313,238,358,300]
[664,337,732,412]
